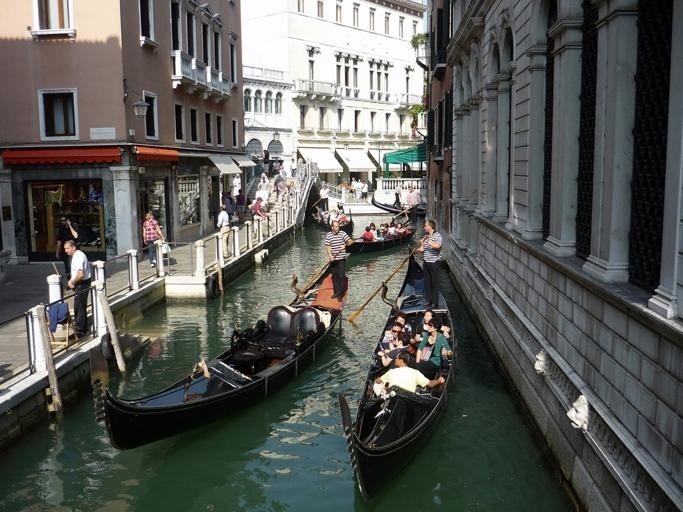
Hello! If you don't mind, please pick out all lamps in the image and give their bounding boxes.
[130,91,150,121]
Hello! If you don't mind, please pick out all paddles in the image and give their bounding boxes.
[349,235,428,323]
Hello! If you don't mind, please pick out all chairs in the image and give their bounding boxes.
[257,306,319,358]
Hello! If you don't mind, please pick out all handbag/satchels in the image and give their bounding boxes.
[161,241,171,255]
[420,346,432,362]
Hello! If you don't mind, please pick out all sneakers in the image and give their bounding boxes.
[151,260,156,268]
[69,330,86,339]
[331,293,342,302]
[423,301,439,311]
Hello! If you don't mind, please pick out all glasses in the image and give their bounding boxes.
[392,329,401,334]
[428,323,434,328]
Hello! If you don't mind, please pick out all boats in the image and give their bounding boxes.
[369,193,426,217]
[338,242,458,504]
[100,257,348,451]
[313,207,354,237]
[341,227,416,255]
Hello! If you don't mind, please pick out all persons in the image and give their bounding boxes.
[222,157,303,238]
[407,186,421,228]
[338,177,367,203]
[216,205,230,258]
[325,221,353,299]
[411,220,442,309]
[55,214,78,281]
[373,311,452,407]
[142,213,164,267]
[311,184,349,225]
[63,240,91,339]
[363,223,409,242]
[392,184,403,208]
